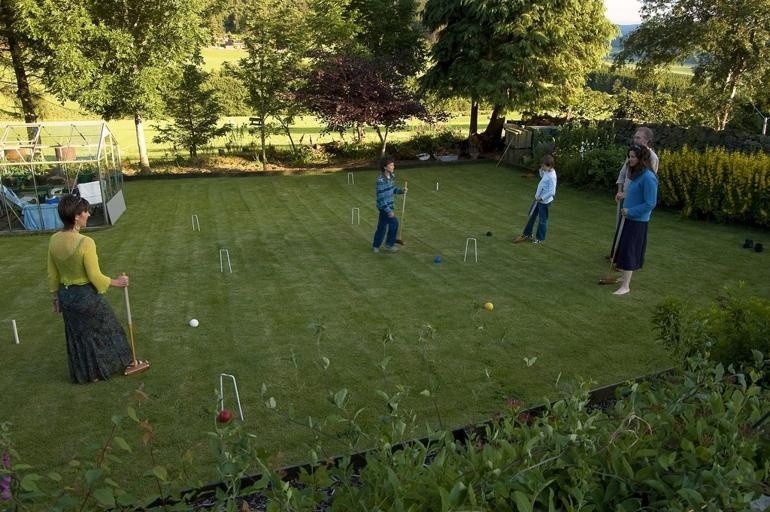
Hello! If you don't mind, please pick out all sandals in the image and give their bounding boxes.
[126,361,148,369]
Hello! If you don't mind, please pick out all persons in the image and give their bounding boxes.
[610,127,659,294]
[372,157,408,253]
[519,154,557,244]
[47,196,133,384]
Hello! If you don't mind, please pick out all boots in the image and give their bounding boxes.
[373,245,400,253]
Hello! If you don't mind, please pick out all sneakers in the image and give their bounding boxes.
[524,233,533,239]
[534,239,543,243]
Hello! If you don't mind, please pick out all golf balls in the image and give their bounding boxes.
[487,231,493,235]
[190,319,199,327]
[220,410,231,421]
[434,256,441,262]
[484,302,494,310]
